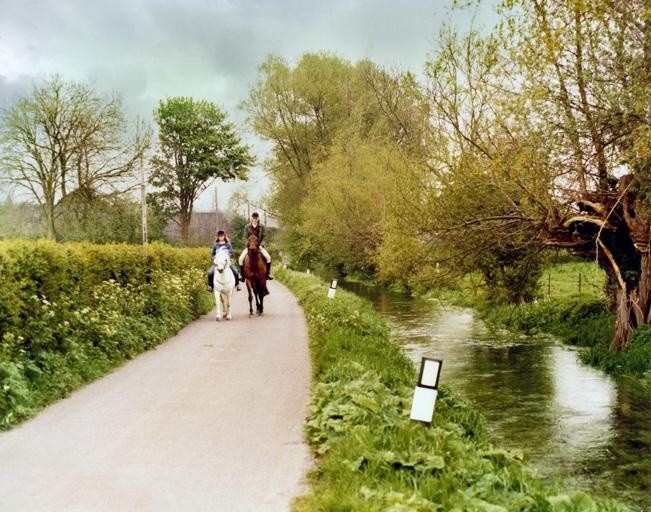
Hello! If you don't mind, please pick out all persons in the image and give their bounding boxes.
[206,230,241,293]
[237,212,275,282]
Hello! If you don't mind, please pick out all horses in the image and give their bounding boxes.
[239,228,269,318]
[210,245,237,323]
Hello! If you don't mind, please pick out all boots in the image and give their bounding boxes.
[265,263,274,281]
[238,265,246,282]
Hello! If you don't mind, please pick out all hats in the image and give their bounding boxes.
[217,230,225,234]
[252,212,259,217]
